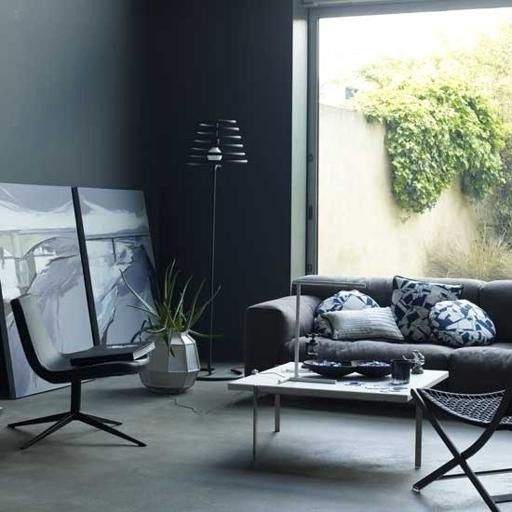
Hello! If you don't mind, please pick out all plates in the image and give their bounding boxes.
[304,357,358,376]
[354,363,390,378]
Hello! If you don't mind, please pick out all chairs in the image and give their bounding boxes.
[7,294,147,450]
[411,377,512,512]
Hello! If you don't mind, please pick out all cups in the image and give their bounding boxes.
[391,358,411,386]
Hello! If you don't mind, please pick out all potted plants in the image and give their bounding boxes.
[119,260,224,395]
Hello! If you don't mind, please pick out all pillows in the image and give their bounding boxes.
[321,306,405,342]
[314,289,380,336]
[429,299,496,347]
[390,275,464,343]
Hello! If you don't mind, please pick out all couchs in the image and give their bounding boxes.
[245,274,512,393]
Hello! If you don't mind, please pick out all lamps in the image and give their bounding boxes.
[185,119,247,379]
